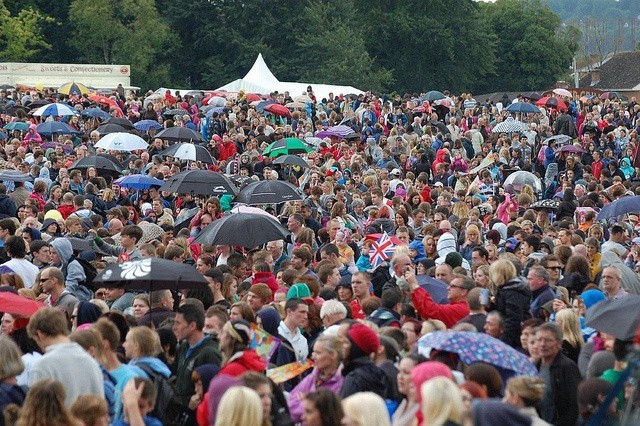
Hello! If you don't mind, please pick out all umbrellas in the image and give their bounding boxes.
[3,122,29,131]
[526,198,560,209]
[264,104,291,117]
[67,155,124,178]
[112,174,165,202]
[415,330,538,378]
[92,132,150,151]
[542,134,572,148]
[229,180,305,206]
[230,206,281,224]
[199,104,215,112]
[95,123,127,134]
[586,296,640,341]
[272,154,310,169]
[594,194,640,225]
[552,145,586,154]
[27,100,50,107]
[145,93,163,100]
[262,136,315,158]
[163,109,186,116]
[535,97,568,111]
[245,93,260,101]
[599,91,628,101]
[421,90,446,101]
[79,108,105,117]
[92,258,210,329]
[0,168,35,183]
[32,103,79,117]
[490,121,530,133]
[0,84,15,89]
[293,95,312,103]
[284,102,309,108]
[502,172,541,192]
[207,96,227,107]
[206,106,224,116]
[552,88,572,98]
[344,135,360,139]
[188,212,294,252]
[40,142,72,153]
[88,96,119,107]
[519,91,542,100]
[158,142,215,166]
[95,153,124,170]
[316,132,343,140]
[430,121,450,136]
[133,119,163,131]
[416,274,449,304]
[100,117,133,127]
[36,121,80,136]
[153,126,205,143]
[5,105,31,117]
[364,233,408,246]
[507,102,540,113]
[327,125,355,136]
[0,133,10,141]
[57,81,90,95]
[432,104,451,115]
[249,99,264,106]
[158,169,239,196]
[1,292,48,318]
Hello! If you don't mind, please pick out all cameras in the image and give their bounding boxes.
[403,265,409,277]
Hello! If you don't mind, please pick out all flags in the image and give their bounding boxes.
[367,233,394,270]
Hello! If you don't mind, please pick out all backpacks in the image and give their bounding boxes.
[62,255,99,292]
[75,209,93,232]
[136,361,184,426]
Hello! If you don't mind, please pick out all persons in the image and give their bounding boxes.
[86,289,135,330]
[600,101,640,139]
[189,194,233,228]
[521,308,592,426]
[0,183,92,299]
[540,216,639,303]
[56,93,89,111]
[291,110,317,137]
[374,271,420,426]
[510,95,529,104]
[358,125,431,203]
[205,253,373,426]
[0,91,26,105]
[163,86,204,109]
[0,313,206,426]
[524,109,576,135]
[35,154,76,182]
[0,131,29,173]
[432,133,501,196]
[142,100,163,120]
[395,234,543,272]
[261,89,292,104]
[370,192,527,234]
[406,271,475,327]
[24,124,43,143]
[135,291,179,331]
[278,202,371,273]
[558,154,599,217]
[203,116,264,180]
[173,287,221,328]
[496,134,542,171]
[586,129,640,203]
[28,137,48,169]
[343,93,421,125]
[1,114,41,124]
[468,260,587,330]
[418,101,432,125]
[449,90,492,133]
[116,82,144,119]
[409,363,565,426]
[263,167,312,186]
[45,148,56,160]
[492,99,508,123]
[77,179,130,257]
[187,104,201,128]
[251,116,292,140]
[59,135,92,145]
[246,107,264,121]
[579,333,640,425]
[158,115,206,127]
[305,158,377,202]
[311,92,343,125]
[55,144,64,154]
[132,197,202,262]
[224,90,247,125]
[568,97,599,145]
[17,87,57,101]
[41,267,92,343]
[310,140,384,158]
[542,147,561,198]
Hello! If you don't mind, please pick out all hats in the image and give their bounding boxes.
[612,176,622,181]
[397,136,402,141]
[507,374,546,401]
[105,209,123,217]
[223,321,250,345]
[625,214,639,223]
[347,323,380,357]
[433,182,443,188]
[505,241,522,254]
[389,169,400,176]
[145,163,154,173]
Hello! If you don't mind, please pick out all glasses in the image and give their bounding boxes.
[39,278,49,283]
[600,275,615,281]
[70,314,76,320]
[450,285,466,289]
[433,219,444,223]
[94,292,106,298]
[545,266,562,271]
